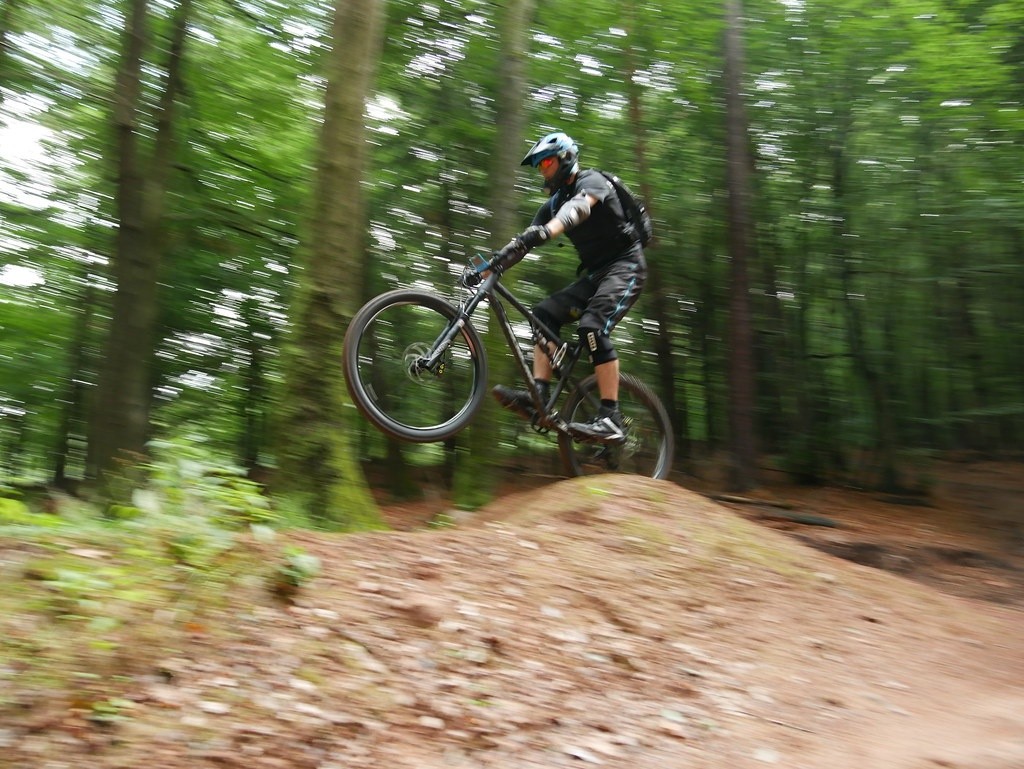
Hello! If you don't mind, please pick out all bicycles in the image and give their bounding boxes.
[343,227,676,484]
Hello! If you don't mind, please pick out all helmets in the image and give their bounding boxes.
[520,133,579,197]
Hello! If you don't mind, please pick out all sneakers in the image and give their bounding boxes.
[568,410,625,445]
[493,383,549,419]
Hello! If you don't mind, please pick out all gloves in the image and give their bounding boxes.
[522,224,550,249]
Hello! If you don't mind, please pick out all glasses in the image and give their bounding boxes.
[539,158,559,170]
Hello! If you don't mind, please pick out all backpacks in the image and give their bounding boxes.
[550,170,655,247]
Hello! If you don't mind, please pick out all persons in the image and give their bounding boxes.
[462,132,647,445]
